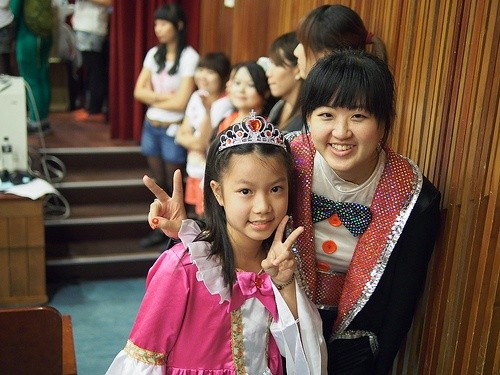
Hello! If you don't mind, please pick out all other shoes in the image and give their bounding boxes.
[27,124,49,136]
[139,231,166,248]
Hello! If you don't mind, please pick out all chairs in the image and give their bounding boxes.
[0,305,77,375]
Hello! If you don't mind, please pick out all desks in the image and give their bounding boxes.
[0,194,48,308]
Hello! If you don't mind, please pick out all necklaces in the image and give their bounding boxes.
[256,269,263,275]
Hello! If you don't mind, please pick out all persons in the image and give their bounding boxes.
[133,2,389,249]
[0,0,110,135]
[105,108,328,375]
[143,50,443,375]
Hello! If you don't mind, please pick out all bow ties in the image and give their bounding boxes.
[311,192,372,238]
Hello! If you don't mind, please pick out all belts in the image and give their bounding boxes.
[145,114,184,128]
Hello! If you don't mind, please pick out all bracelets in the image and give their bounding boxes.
[272,272,295,290]
